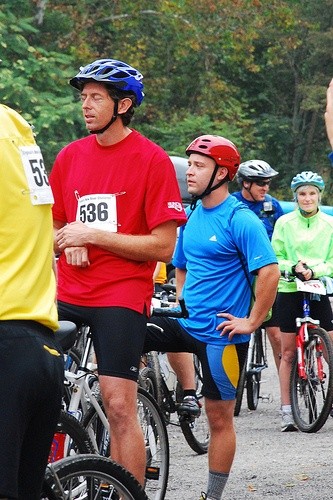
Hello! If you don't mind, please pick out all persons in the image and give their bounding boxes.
[228,159,285,373]
[0,104,64,500]
[44,59,188,500]
[271,170,333,431]
[132,136,280,500]
[323,76,333,168]
[92,217,200,416]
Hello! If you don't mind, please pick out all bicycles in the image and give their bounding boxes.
[36,277,270,500]
[277,268,333,433]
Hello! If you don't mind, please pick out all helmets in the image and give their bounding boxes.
[237,160,279,186]
[186,135,240,182]
[291,171,325,193]
[70,59,145,108]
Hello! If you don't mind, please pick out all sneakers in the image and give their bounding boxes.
[280,412,299,432]
[177,396,202,418]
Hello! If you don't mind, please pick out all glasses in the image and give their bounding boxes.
[256,181,271,186]
